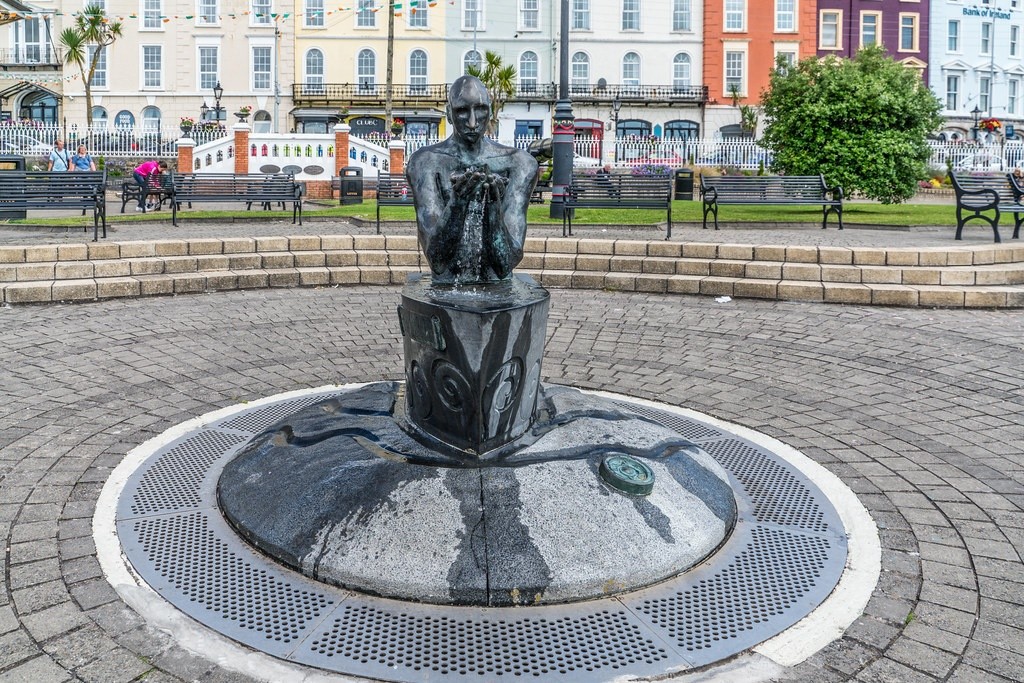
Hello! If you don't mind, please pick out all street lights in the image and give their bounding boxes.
[213,80,223,132]
[612,94,623,167]
[970,103,983,145]
[199,101,209,119]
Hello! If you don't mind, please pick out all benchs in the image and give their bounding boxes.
[699,172,844,231]
[121,172,303,226]
[331,170,676,238]
[0,165,108,243]
[947,169,1024,243]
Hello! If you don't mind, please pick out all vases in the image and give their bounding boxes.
[390,128,404,140]
[233,112,251,124]
[180,126,192,138]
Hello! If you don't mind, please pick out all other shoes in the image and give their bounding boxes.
[137,203,155,211]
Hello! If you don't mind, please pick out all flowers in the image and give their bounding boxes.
[239,104,252,112]
[391,119,407,129]
[181,116,195,125]
[981,116,1002,132]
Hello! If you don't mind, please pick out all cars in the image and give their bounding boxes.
[0,134,212,156]
[537,151,605,168]
[953,152,1008,173]
[624,149,683,168]
[693,146,776,169]
[1009,159,1024,175]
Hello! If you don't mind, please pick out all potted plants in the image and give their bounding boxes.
[335,108,350,124]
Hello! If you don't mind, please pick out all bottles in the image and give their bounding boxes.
[402,185,408,200]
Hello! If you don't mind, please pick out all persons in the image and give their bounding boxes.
[48,140,72,201]
[71,145,96,201]
[596,164,620,198]
[405,76,539,288]
[1014,168,1024,206]
[132,161,167,212]
[147,170,166,211]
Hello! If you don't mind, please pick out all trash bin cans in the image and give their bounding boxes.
[340,166,364,206]
[0,155,27,219]
[675,168,694,200]
